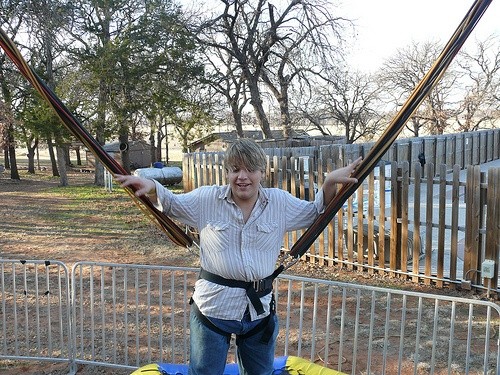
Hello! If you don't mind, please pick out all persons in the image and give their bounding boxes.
[113,138,363,375]
